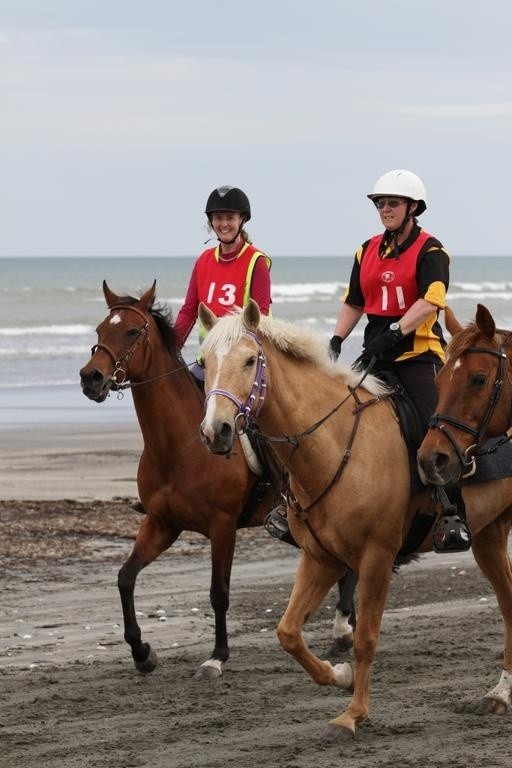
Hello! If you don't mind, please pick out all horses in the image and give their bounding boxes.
[416,304,511,487]
[79,276,357,680]
[192,298,511,739]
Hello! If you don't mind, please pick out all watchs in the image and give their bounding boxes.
[388,323,408,339]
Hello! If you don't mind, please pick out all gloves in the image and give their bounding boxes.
[329,336,343,361]
[367,328,403,353]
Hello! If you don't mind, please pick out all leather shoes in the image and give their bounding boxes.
[130,501,146,514]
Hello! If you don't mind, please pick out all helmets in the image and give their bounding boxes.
[367,169,427,216]
[205,185,251,222]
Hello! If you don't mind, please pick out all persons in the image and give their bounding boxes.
[267,167,474,551]
[130,184,273,516]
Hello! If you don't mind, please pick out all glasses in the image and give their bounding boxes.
[374,200,409,209]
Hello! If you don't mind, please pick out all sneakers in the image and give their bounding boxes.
[442,529,469,542]
[271,517,288,532]
